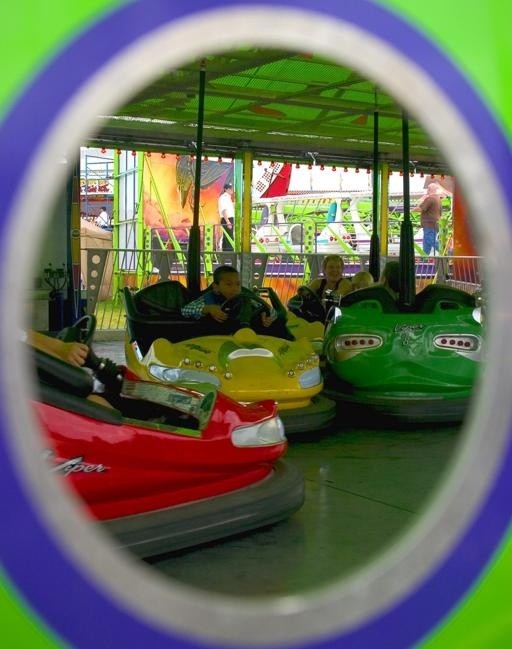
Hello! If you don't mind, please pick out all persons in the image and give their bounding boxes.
[96,206,109,229]
[218,184,235,250]
[413,183,447,263]
